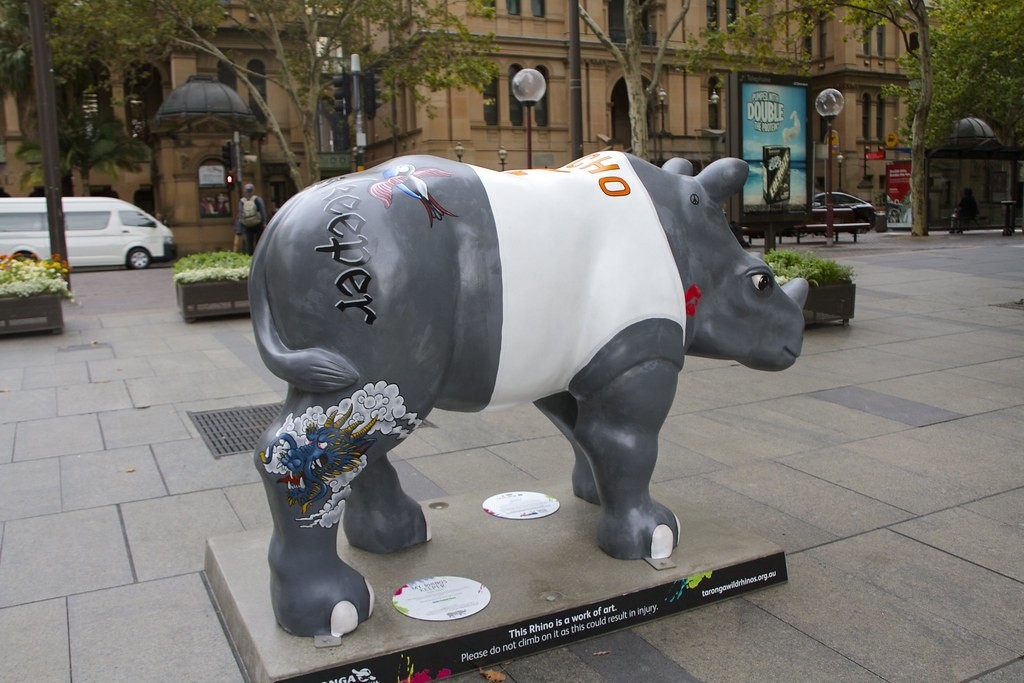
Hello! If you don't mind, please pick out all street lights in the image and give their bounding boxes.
[657,88,666,134]
[836,153,844,193]
[512,67,546,168]
[814,88,845,245]
[711,89,719,130]
[455,140,463,161]
[497,145,508,171]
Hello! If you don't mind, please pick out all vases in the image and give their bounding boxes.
[802,280,856,329]
[0,293,65,336]
[174,281,251,323]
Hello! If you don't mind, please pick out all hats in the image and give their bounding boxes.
[243,184,254,193]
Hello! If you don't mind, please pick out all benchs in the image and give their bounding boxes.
[941,216,990,235]
[741,227,784,244]
[793,209,871,243]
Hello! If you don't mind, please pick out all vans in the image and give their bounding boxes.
[0,197,174,269]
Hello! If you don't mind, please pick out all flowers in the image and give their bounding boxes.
[757,246,858,286]
[0,250,76,304]
[168,249,252,284]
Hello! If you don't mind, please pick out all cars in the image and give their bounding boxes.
[795,191,875,237]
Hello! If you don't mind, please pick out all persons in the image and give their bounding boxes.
[949,188,977,233]
[235,183,266,255]
[209,192,229,214]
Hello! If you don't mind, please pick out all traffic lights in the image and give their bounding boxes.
[220,140,234,190]
[332,67,349,118]
[364,64,382,119]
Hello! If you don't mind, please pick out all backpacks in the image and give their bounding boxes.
[240,196,261,227]
